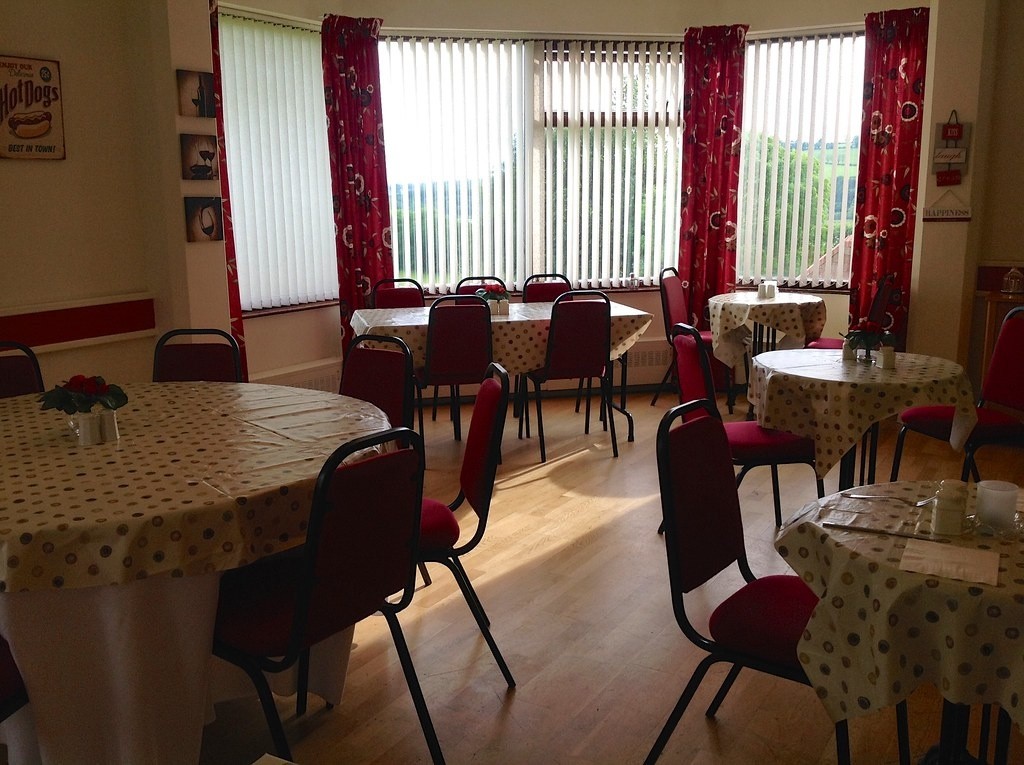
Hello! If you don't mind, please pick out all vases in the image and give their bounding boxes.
[858,349,877,361]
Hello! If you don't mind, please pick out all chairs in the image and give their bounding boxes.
[0,267,1024,765]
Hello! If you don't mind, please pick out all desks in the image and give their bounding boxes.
[708,292,827,422]
[747,348,978,492]
[774,480,1024,765]
[980,291,1024,392]
[0,381,399,765]
[349,299,654,443]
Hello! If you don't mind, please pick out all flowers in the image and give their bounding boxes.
[838,319,896,351]
[36,375,129,414]
[479,283,511,304]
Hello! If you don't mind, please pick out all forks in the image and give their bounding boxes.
[841,493,936,507]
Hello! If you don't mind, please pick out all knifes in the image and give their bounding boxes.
[823,521,952,544]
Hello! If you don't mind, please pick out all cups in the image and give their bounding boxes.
[78,410,120,445]
[876,347,896,368]
[758,285,775,298]
[930,478,969,535]
[488,300,509,315]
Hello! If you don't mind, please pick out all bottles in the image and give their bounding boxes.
[1002,264,1024,293]
